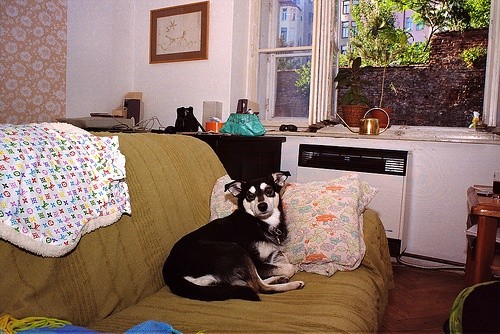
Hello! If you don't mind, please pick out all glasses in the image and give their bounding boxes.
[279,124,297,131]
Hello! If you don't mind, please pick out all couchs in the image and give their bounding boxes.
[0,122,395,334]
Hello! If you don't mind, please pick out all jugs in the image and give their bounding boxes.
[335,108,389,135]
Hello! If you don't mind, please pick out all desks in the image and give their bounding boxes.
[465,185,500,286]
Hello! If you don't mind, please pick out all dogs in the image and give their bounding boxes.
[162,170,305,303]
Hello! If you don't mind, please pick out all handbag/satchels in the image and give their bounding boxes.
[220,99,267,137]
[175,107,206,132]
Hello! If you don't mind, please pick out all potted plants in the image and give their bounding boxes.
[334,57,374,127]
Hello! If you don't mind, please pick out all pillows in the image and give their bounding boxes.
[209,173,380,278]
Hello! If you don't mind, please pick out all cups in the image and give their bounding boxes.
[492,172,500,198]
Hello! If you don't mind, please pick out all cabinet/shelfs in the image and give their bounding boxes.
[151,130,286,179]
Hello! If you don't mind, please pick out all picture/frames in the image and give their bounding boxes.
[149,1,210,64]
